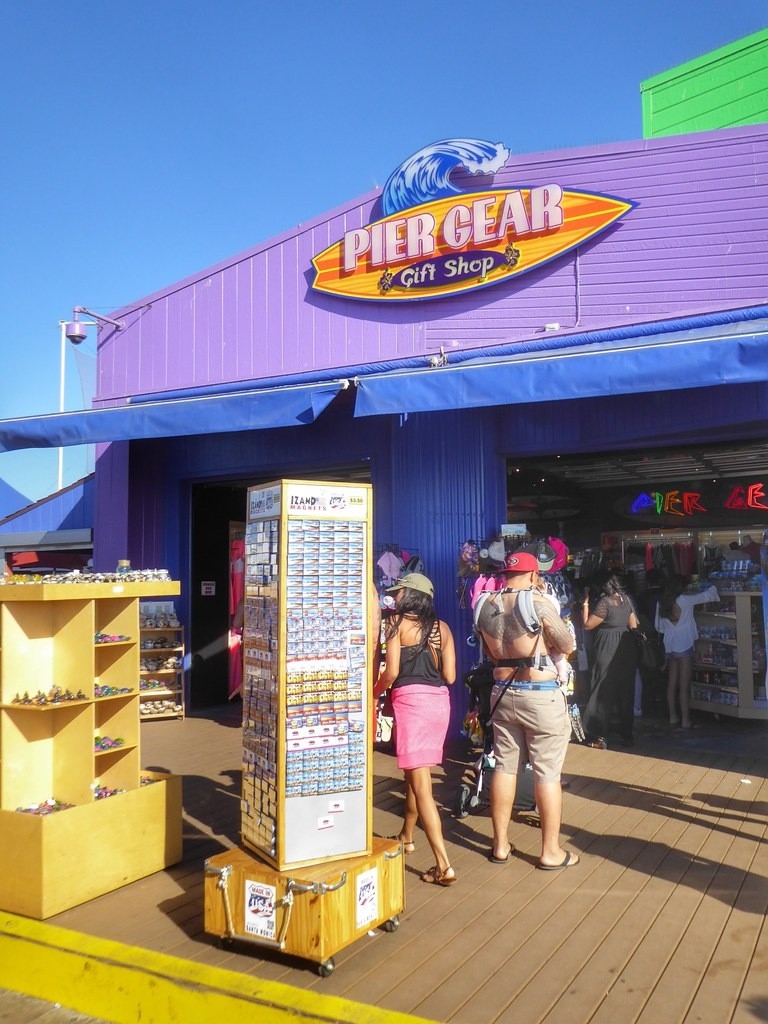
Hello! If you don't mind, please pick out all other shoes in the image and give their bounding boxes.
[588,740,607,749]
[634,707,643,716]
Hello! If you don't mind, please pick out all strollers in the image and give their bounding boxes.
[451,631,540,820]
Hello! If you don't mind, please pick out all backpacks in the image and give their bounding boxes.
[400,554,425,578]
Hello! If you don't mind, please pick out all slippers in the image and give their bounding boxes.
[681,720,701,729]
[491,842,516,864]
[539,850,580,869]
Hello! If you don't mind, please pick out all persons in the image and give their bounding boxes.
[373,572,459,886]
[582,574,637,748]
[472,552,580,870]
[233,597,244,671]
[642,568,666,627]
[655,573,721,727]
[373,583,382,661]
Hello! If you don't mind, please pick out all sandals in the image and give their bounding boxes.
[387,835,416,854]
[420,866,456,884]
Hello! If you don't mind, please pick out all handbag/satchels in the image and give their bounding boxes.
[626,625,651,665]
[477,707,495,754]
[479,754,537,811]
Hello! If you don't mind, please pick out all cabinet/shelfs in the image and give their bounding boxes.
[687,592,768,720]
[139,626,185,722]
[0,581,181,919]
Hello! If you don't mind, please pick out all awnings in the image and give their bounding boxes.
[0,378,350,452]
[354,318,768,417]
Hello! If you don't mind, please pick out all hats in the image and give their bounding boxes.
[481,536,568,574]
[384,573,435,600]
